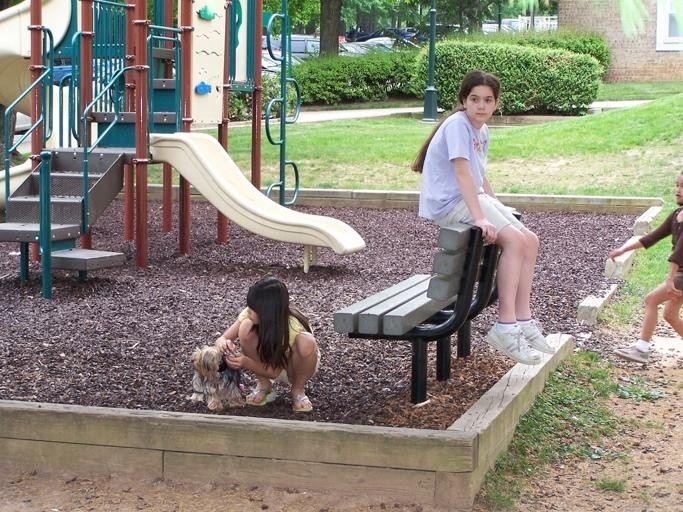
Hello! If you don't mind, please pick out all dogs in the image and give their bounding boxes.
[189,342,247,411]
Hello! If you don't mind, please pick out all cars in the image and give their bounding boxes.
[260,22,517,72]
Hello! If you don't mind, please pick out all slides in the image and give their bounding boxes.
[147,132,366,256]
[0,0,113,207]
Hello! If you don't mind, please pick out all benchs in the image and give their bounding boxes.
[334,207,522,405]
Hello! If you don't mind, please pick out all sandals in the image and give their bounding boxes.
[292,395,312,412]
[246,381,276,406]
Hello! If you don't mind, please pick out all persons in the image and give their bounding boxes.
[212,276,319,412]
[409,69,555,367]
[604,168,682,366]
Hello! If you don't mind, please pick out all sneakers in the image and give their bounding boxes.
[613,345,649,363]
[487,319,556,365]
